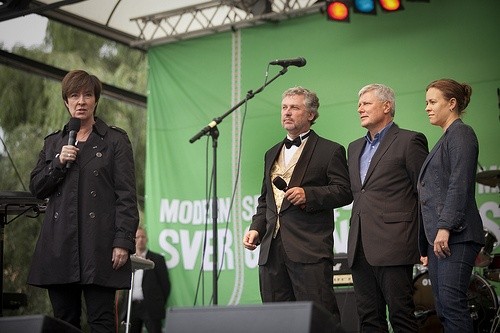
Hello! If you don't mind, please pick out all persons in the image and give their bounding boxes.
[125,228,171,333]
[243,86,354,333]
[415,79,486,333]
[27,69,140,332]
[346,83,430,333]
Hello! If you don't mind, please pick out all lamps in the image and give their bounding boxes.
[326,0,404,23]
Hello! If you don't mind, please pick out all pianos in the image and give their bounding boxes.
[0,191,51,317]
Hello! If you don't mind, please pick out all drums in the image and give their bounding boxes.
[473,226,498,266]
[483,252,500,283]
[409,270,500,333]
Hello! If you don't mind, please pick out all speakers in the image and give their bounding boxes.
[162,301,340,333]
[0,315,82,333]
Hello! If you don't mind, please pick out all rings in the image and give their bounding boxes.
[441,245,444,249]
[68,154,70,157]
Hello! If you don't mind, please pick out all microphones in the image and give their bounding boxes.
[66,118,81,168]
[273,176,306,210]
[269,56,306,68]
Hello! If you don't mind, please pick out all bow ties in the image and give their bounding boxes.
[284,131,310,149]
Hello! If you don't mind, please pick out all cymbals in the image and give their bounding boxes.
[475,170,500,189]
